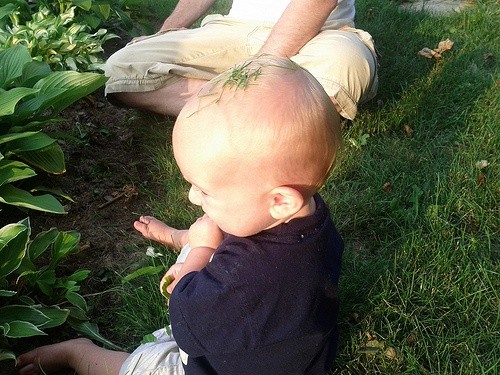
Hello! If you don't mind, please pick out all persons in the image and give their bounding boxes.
[104,0,380,127]
[16,54,345,375]
